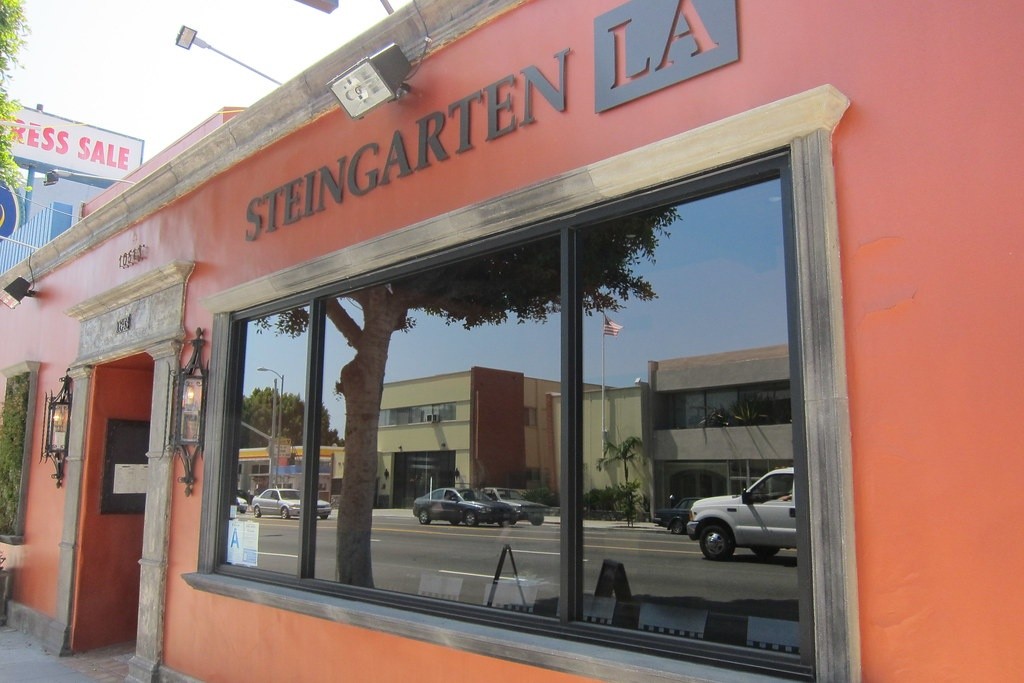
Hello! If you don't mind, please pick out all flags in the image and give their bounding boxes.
[603,314,624,336]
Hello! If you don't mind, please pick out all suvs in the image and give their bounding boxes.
[686,466,797,562]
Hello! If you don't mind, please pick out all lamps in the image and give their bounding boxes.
[328,41,414,123]
[157,326,209,496]
[175,24,283,85]
[43,169,134,187]
[38,368,72,489]
[0,276,36,309]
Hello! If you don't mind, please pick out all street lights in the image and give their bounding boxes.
[257,366,284,488]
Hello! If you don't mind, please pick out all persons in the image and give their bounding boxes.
[492,493,497,500]
[444,492,452,499]
[776,481,792,501]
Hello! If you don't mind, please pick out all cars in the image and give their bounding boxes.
[653,496,708,534]
[250,487,332,520]
[481,487,551,526]
[236,496,248,514]
[412,486,515,527]
